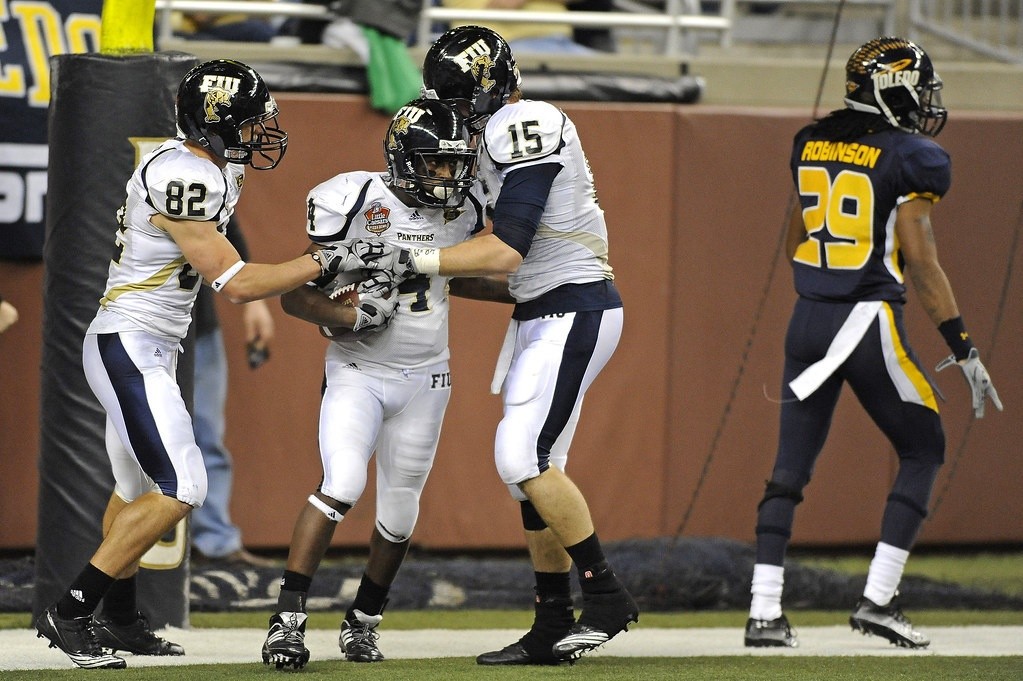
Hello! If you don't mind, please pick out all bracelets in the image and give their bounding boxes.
[309,251,326,276]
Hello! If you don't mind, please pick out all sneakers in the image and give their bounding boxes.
[92,609,186,656]
[848,594,930,649]
[261,611,310,671]
[338,609,385,663]
[33,602,127,671]
[743,612,799,648]
[552,569,642,661]
[475,614,578,667]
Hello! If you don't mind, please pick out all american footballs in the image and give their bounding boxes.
[319,282,392,341]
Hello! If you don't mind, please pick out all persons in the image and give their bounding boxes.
[391,25,641,664]
[190,207,279,573]
[742,34,1007,648]
[260,95,516,674]
[34,59,394,669]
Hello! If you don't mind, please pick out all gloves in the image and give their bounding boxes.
[934,348,1004,419]
[354,288,403,331]
[321,238,392,270]
[357,245,416,298]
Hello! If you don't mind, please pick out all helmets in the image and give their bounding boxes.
[174,58,288,171]
[423,26,523,135]
[382,98,477,208]
[845,36,948,138]
